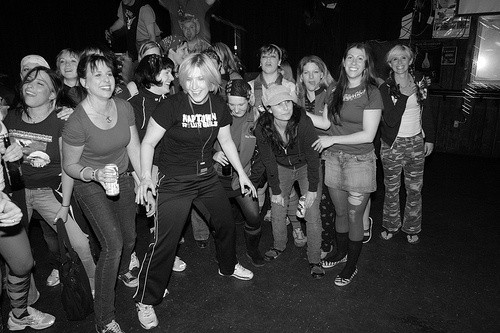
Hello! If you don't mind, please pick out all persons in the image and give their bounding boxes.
[377,45,435,243]
[0,0,385,333]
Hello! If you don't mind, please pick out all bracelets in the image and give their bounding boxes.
[91,168,100,181]
[80,166,93,183]
[62,203,70,207]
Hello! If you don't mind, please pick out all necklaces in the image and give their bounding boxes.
[87,94,112,125]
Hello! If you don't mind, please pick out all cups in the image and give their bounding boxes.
[221,161,232,177]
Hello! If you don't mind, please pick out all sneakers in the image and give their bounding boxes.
[218,262,254,281]
[7,306,56,330]
[135,301,159,329]
[45,268,61,286]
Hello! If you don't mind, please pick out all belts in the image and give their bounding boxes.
[25,186,52,190]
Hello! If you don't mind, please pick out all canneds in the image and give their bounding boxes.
[296,196,306,217]
[105,163,121,197]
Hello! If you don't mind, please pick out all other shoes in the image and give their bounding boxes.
[321,252,348,268]
[309,263,325,280]
[171,255,187,272]
[245,248,266,267]
[263,209,272,222]
[263,247,288,262]
[196,239,208,249]
[292,227,307,248]
[96,320,126,333]
[334,264,359,287]
[118,271,139,288]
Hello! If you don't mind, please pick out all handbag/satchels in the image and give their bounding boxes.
[55,218,95,321]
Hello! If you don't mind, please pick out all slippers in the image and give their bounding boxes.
[405,232,421,244]
[378,229,400,240]
[362,217,373,243]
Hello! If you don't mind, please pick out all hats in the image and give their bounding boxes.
[225,79,251,100]
[261,85,296,108]
